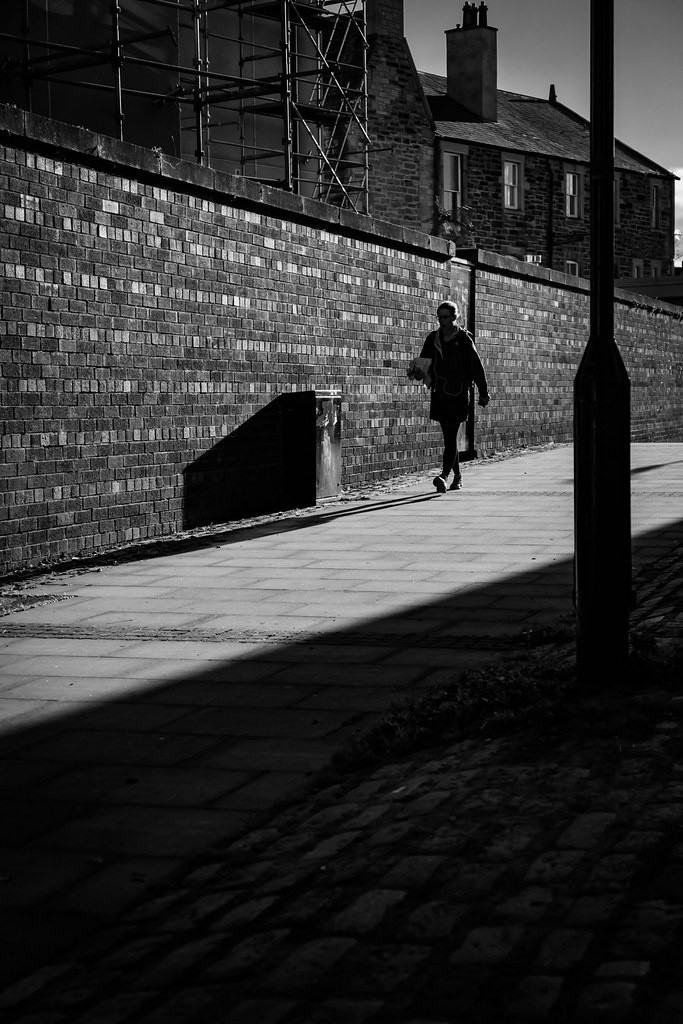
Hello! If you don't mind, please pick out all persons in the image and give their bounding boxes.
[406,301,490,493]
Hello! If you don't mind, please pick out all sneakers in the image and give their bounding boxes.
[450,474,463,490]
[433,474,448,493]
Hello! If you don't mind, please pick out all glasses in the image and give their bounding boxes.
[435,315,451,320]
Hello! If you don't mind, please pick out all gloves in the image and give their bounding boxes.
[478,392,490,408]
[414,368,425,381]
